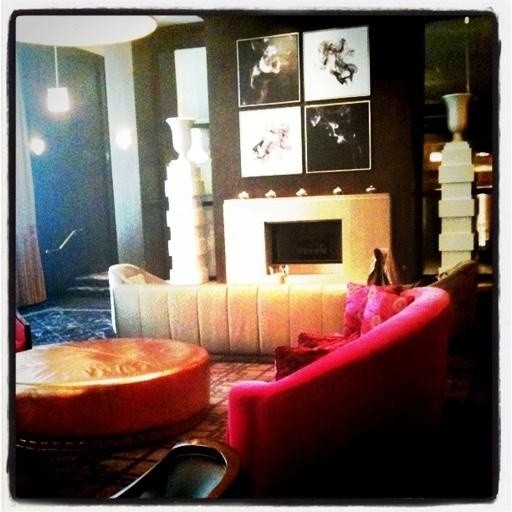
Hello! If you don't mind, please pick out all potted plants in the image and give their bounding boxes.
[235,33,302,109]
[304,99,373,176]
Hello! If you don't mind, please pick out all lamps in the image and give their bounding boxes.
[15,308,34,350]
[16,436,102,502]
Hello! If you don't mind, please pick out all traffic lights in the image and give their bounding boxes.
[125,272,147,286]
[273,280,419,380]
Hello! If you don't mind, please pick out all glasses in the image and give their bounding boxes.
[43,31,71,116]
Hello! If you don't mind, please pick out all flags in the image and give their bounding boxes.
[163,116,196,160]
[442,93,473,140]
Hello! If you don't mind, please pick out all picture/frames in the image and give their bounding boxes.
[104,433,245,501]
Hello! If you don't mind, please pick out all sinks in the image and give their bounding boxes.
[103,249,485,363]
[227,281,451,479]
